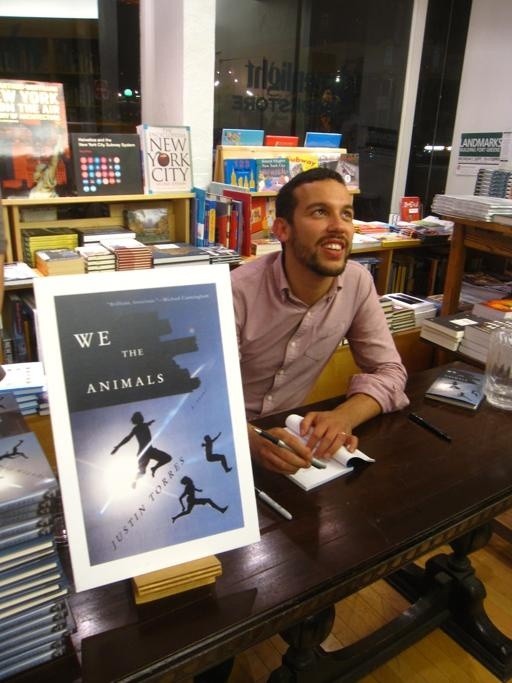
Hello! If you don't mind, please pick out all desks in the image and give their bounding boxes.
[57,362,512,683]
[436,215,512,367]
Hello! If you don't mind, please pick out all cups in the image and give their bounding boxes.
[483,322,512,410]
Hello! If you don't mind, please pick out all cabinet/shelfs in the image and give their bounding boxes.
[1,192,195,291]
[215,145,453,295]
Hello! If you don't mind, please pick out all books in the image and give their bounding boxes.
[1,80,512,683]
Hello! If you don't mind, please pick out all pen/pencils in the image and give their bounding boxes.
[250,426,327,471]
[251,484,293,520]
[402,406,452,443]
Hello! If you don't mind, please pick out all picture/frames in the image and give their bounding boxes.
[33,264,261,594]
